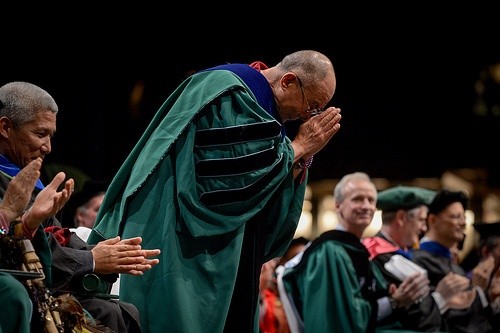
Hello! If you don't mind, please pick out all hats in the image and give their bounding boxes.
[75,180,109,207]
[429,190,469,215]
[376,184,439,213]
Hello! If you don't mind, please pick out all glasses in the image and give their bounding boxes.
[296,76,323,115]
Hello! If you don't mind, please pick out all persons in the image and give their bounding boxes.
[283,171,428,333]
[0,158,75,332]
[1,80,160,332]
[254,235,312,332]
[85,50,341,331]
[471,218,500,333]
[68,193,122,300]
[351,184,477,333]
[406,188,495,333]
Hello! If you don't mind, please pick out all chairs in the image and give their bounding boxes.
[275,240,314,333]
